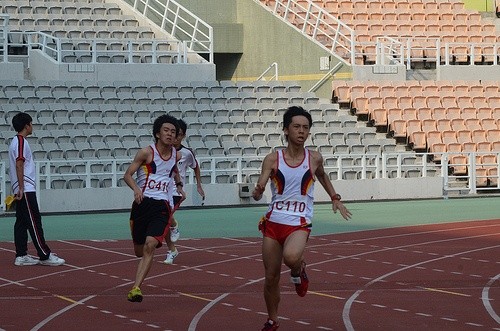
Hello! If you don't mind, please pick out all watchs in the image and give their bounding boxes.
[175,182,184,187]
[331,194,342,201]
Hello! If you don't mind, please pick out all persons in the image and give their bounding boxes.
[123,114,187,304]
[162,119,205,266]
[251,105,353,331]
[8,112,65,267]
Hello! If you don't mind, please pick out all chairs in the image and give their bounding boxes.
[0,80,500,211]
[0,0,184,64]
[260,0,500,69]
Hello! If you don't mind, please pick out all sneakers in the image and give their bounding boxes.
[127,288,143,302]
[163,247,179,263]
[290,260,309,297]
[260,319,281,330]
[39,252,65,266]
[170,220,180,242]
[14,254,39,266]
